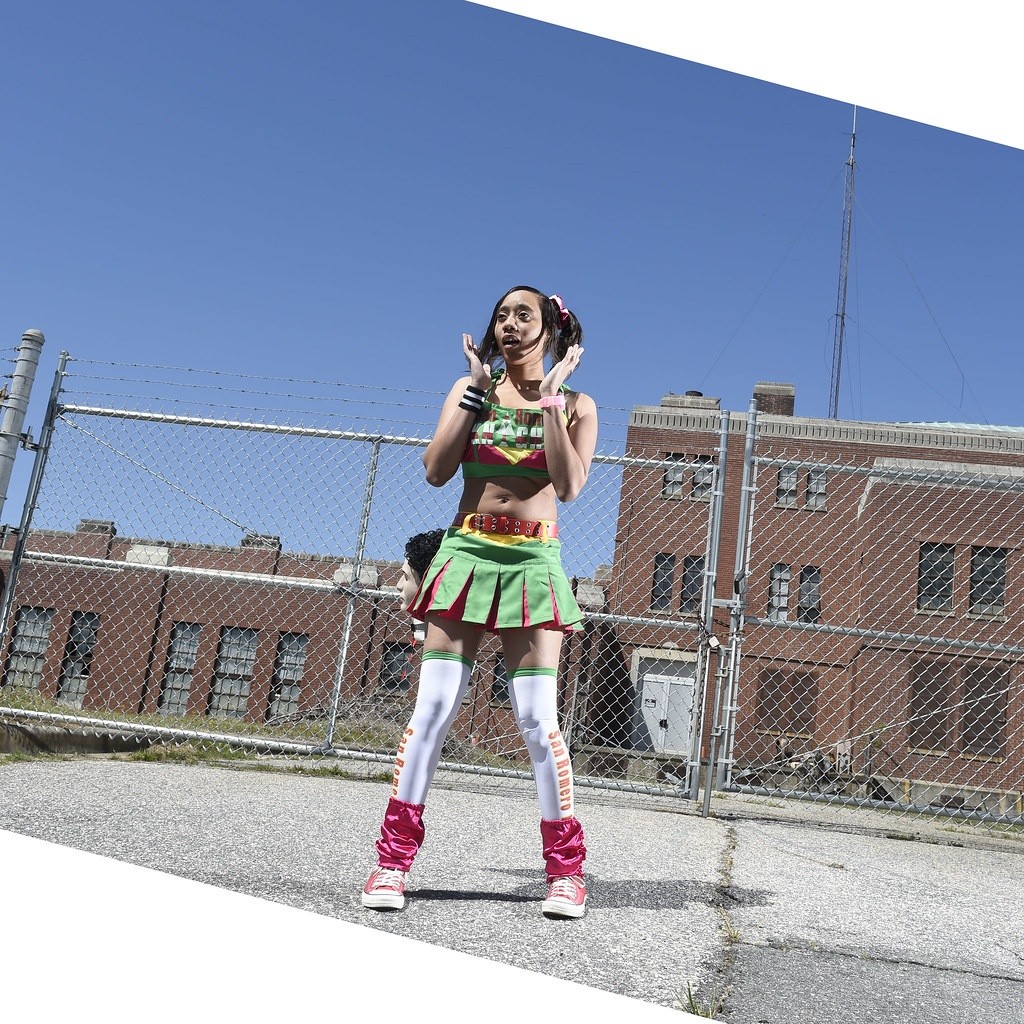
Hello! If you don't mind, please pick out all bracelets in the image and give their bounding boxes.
[539,395,566,411]
[458,385,487,414]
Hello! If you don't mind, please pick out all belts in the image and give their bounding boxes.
[452,511,558,539]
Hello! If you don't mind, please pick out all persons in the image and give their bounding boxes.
[361,285,598,918]
[397,528,448,612]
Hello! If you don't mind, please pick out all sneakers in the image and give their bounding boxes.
[543,875,588,917]
[363,865,409,909]
